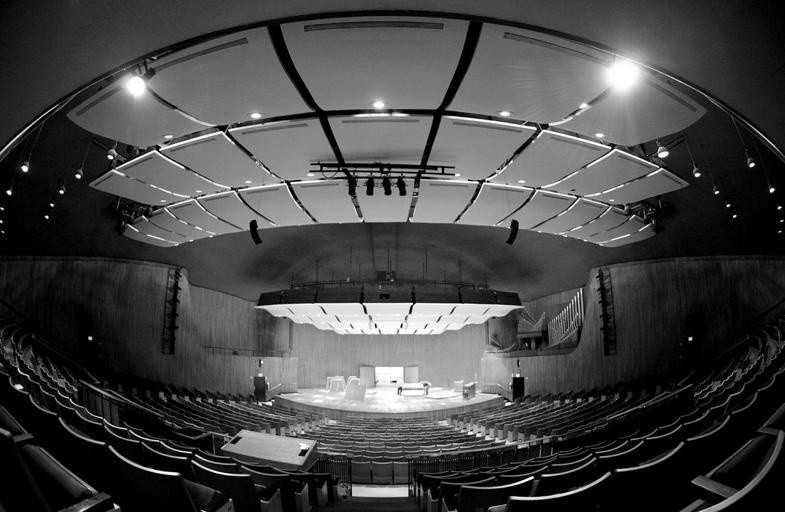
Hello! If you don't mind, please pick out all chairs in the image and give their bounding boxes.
[0,331,785,512]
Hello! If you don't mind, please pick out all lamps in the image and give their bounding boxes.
[649,110,758,195]
[346,178,408,197]
[1,106,128,196]
[249,220,263,246]
[504,219,519,246]
